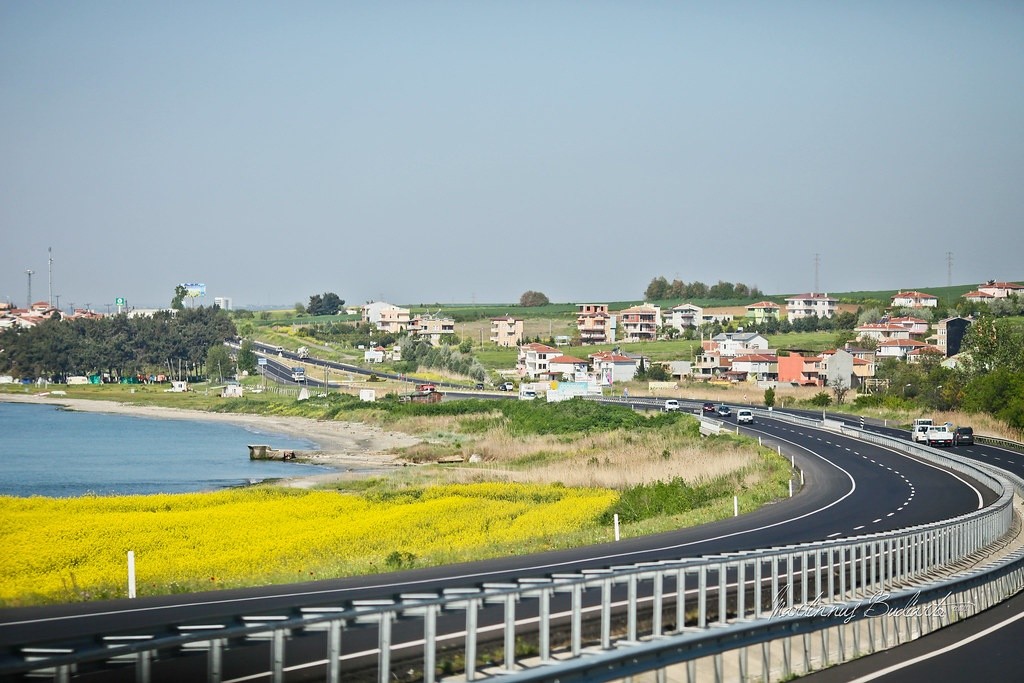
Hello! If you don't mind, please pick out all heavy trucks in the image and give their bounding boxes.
[292,367,304,381]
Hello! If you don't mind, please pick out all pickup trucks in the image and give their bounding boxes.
[926,425,953,447]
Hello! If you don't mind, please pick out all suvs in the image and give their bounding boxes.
[665,400,679,411]
[952,427,974,447]
[737,409,753,425]
[912,425,931,445]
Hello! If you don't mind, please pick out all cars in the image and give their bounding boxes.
[717,405,731,417]
[703,403,714,412]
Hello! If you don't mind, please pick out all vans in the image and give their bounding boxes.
[504,382,513,391]
[914,419,933,428]
